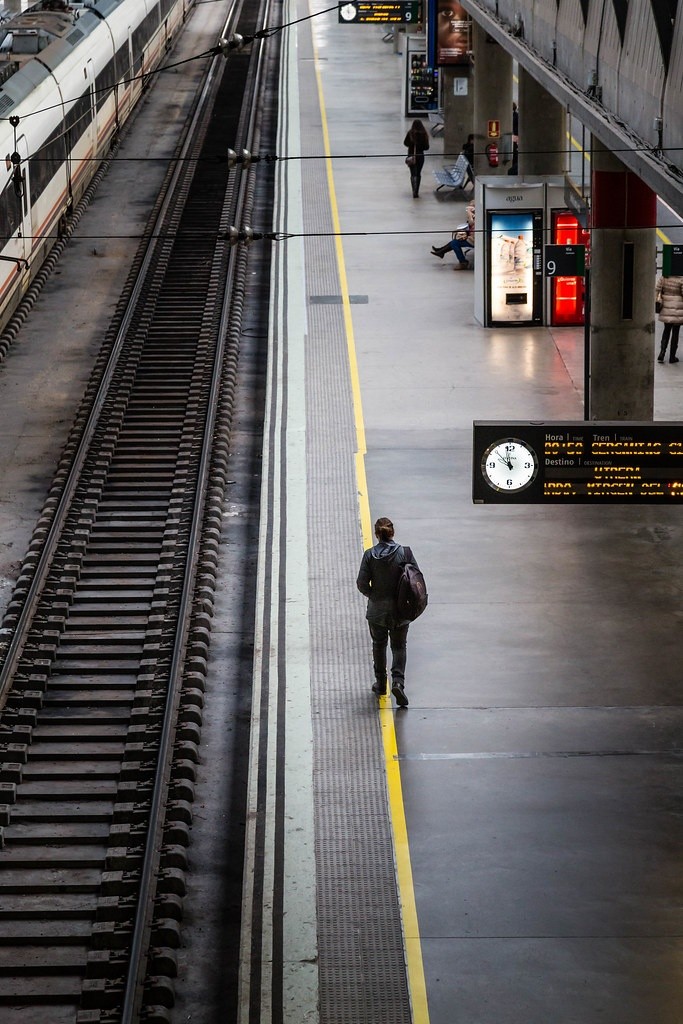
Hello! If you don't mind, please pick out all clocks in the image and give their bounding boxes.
[340,4,357,21]
[481,438,539,493]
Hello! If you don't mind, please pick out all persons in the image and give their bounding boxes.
[437,0,471,64]
[404,119,429,198]
[430,200,475,270]
[356,517,419,705]
[656,275,683,363]
[507,102,518,175]
[462,134,474,170]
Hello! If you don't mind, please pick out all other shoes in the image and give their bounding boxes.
[391,682,409,705]
[373,682,386,694]
[454,263,468,269]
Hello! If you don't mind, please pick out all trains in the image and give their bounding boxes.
[0,0,194,335]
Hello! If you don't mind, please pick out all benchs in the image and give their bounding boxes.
[432,153,477,201]
[429,108,445,138]
[381,24,394,42]
[451,224,474,256]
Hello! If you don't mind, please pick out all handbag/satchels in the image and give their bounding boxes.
[457,223,470,230]
[656,277,665,313]
[395,546,427,621]
[405,156,416,166]
[455,232,467,240]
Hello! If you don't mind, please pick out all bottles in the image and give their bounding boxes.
[499,240,508,265]
[509,241,515,265]
[411,54,438,109]
[514,235,526,270]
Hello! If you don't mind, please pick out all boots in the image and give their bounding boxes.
[670,348,679,362]
[432,242,451,251]
[410,176,421,198]
[431,244,452,258]
[658,341,668,363]
